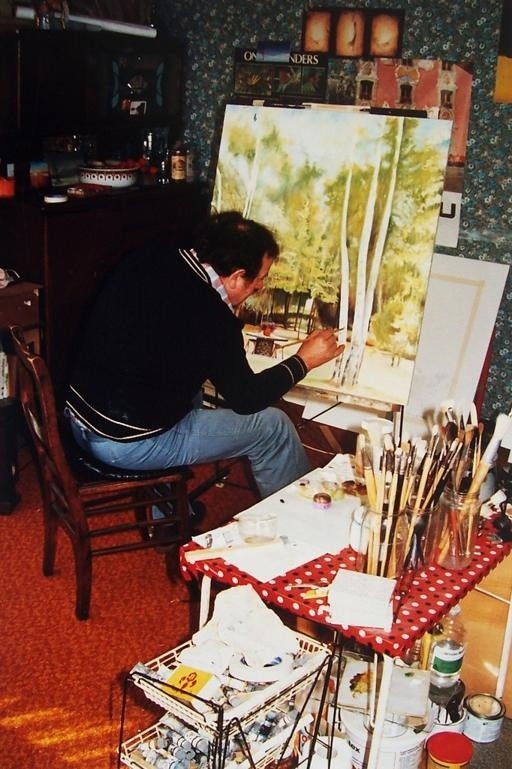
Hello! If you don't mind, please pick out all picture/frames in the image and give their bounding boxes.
[179,452,512,769]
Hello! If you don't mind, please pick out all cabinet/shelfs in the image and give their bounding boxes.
[1,29,209,399]
[118,627,347,769]
[170,140,187,181]
[432,606,466,691]
[350,491,484,579]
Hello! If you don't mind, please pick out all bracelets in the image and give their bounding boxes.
[77,167,140,188]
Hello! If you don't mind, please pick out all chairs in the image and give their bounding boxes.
[150,499,206,541]
[8,324,192,621]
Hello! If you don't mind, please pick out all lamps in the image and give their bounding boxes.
[8,324,192,621]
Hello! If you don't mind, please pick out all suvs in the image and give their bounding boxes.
[338,708,433,768]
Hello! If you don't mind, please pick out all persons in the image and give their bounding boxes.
[53,208,350,524]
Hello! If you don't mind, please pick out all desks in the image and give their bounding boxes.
[179,452,512,769]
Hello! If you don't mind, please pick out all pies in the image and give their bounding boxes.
[354,402,512,578]
[281,328,344,348]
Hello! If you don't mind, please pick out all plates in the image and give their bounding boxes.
[118,627,347,769]
